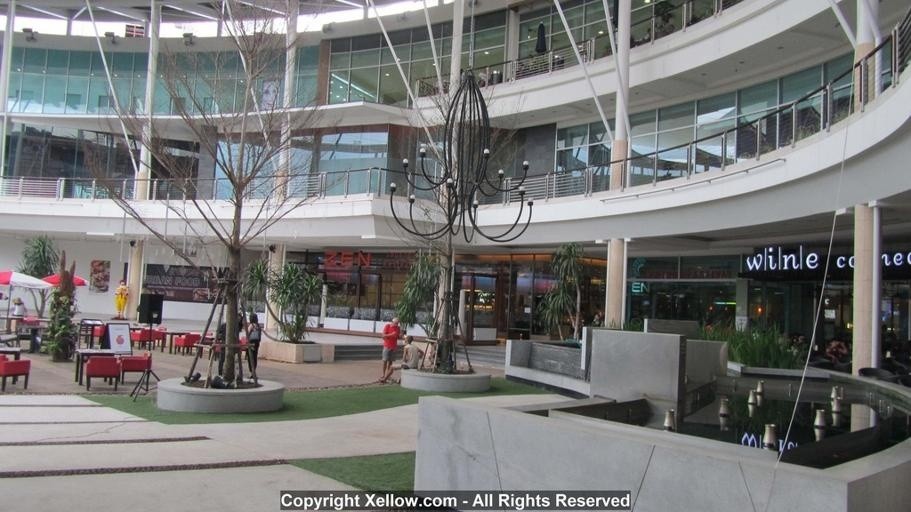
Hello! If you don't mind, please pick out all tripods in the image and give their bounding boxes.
[129,323,161,402]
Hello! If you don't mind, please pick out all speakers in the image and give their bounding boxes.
[139,293,164,325]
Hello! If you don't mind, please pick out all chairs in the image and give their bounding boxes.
[858,365,897,382]
[0,306,258,394]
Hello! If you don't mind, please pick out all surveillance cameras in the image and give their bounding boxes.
[129,241,135,246]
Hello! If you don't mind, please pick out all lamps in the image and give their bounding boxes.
[381,0,538,258]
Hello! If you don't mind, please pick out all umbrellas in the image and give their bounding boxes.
[0,270,55,331]
[41,271,92,289]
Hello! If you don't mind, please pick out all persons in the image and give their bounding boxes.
[10,298,29,316]
[261,79,280,112]
[112,279,129,319]
[216,314,243,375]
[379,335,427,382]
[246,312,261,379]
[430,341,441,366]
[380,317,405,377]
